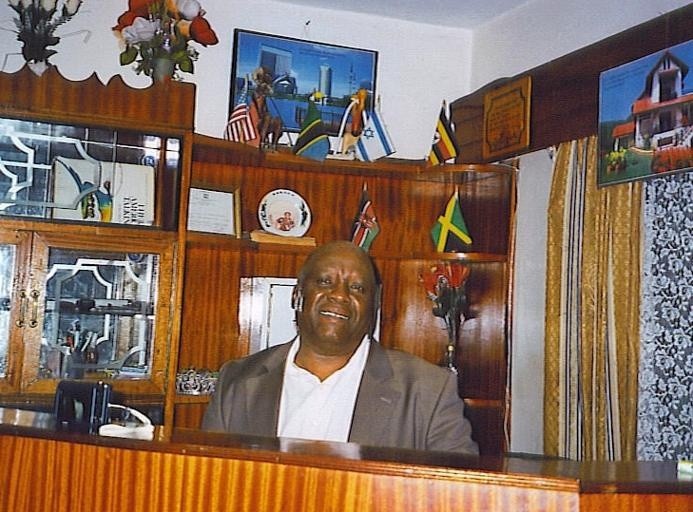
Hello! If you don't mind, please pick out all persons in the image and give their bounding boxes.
[277,212,294,231]
[197,236,480,459]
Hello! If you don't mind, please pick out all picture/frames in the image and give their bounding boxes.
[229,28,378,137]
[596,40,693,189]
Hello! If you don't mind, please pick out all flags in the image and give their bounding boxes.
[428,191,473,255]
[220,85,262,146]
[286,100,332,165]
[353,109,397,162]
[347,189,381,255]
[423,106,459,171]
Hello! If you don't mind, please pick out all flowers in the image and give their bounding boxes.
[4,0,82,40]
[416,262,483,360]
[112,0,220,76]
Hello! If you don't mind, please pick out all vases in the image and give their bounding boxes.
[151,56,176,85]
[19,32,52,66]
[437,345,463,399]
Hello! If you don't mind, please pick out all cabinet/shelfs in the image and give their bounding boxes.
[0,65,516,453]
